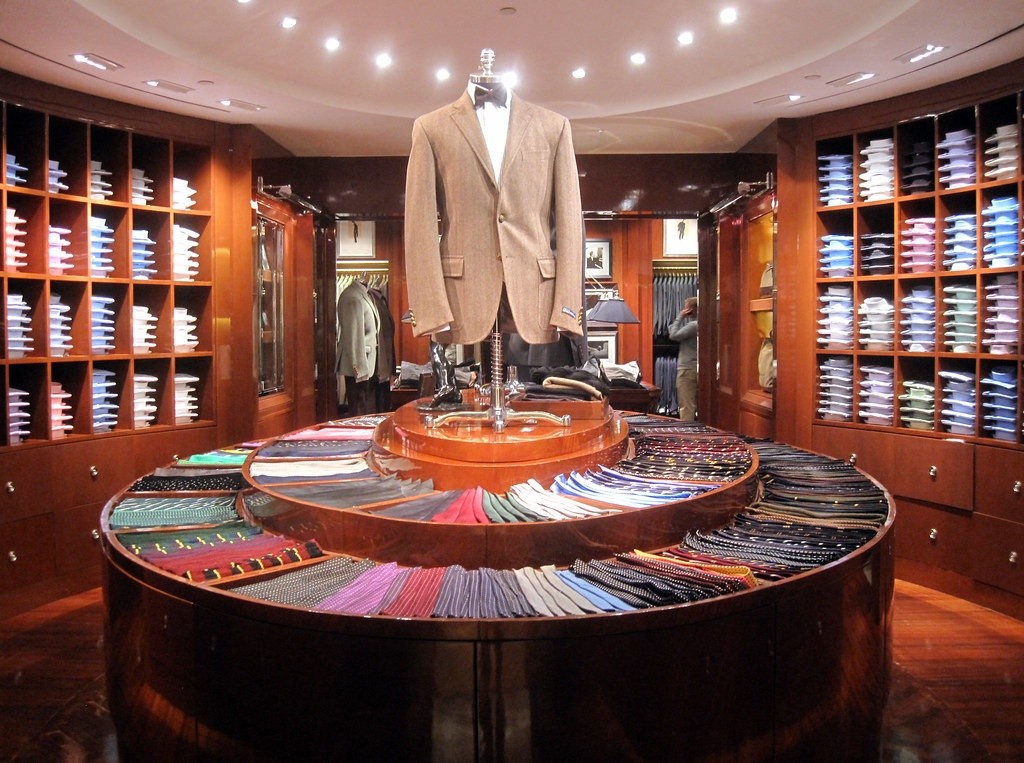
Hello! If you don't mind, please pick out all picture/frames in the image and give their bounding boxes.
[585,238,612,280]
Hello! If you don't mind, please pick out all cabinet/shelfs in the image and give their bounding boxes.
[0,86,319,620]
[698,55,1024,623]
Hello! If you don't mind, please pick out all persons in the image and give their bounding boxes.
[667,297,697,422]
[677,219,685,241]
[404,75,584,345]
[366,286,396,413]
[334,279,381,417]
[587,251,599,268]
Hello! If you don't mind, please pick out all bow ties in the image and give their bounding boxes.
[475,83,507,110]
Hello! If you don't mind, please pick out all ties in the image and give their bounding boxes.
[108,413,888,619]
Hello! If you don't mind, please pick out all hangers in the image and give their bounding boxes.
[336,272,389,284]
[653,267,696,278]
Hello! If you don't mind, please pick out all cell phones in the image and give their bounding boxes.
[687,311,693,316]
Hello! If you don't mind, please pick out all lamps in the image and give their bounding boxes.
[585,288,641,325]
